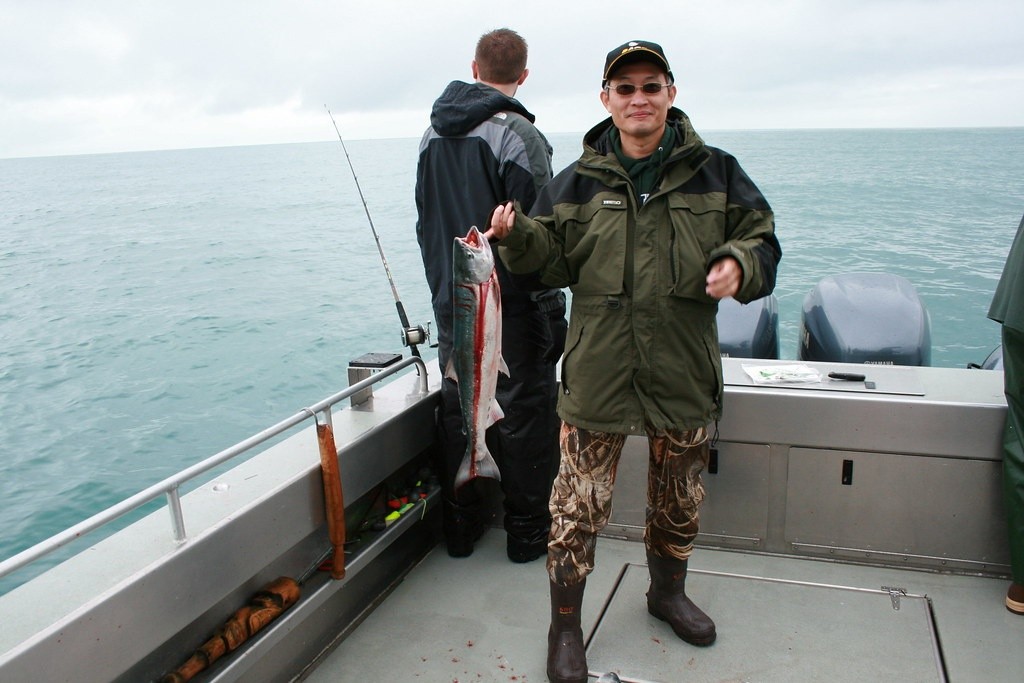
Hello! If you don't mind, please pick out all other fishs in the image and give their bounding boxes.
[443,224,511,494]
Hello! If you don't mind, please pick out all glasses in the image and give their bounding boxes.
[607,82,671,96]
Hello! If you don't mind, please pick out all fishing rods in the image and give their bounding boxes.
[322,102,438,378]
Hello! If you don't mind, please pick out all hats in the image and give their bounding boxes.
[602,40,675,89]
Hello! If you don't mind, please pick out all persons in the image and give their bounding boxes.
[983,220,1024,613]
[409,29,568,564]
[469,40,782,683]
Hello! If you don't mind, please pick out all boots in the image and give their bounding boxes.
[547,577,587,683]
[646,551,717,646]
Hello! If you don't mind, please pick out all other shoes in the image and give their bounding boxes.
[1006,582,1024,615]
[504,511,553,563]
[442,497,497,557]
